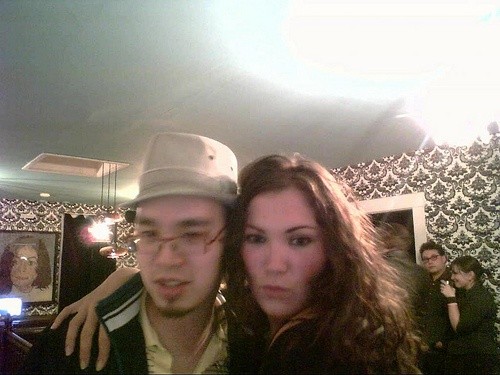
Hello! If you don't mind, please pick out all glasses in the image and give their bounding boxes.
[125,222,227,254]
[422,254,442,263]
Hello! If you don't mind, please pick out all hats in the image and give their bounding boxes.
[120,133,242,207]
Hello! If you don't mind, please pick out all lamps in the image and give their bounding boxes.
[88,162,126,259]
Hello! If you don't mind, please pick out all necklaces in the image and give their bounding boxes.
[144,294,216,369]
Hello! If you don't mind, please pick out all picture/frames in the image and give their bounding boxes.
[0,230,59,307]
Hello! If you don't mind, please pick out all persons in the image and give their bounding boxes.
[420,240,462,375]
[0,236,52,304]
[18,129,270,375]
[50,151,431,375]
[370,222,445,375]
[441,256,497,375]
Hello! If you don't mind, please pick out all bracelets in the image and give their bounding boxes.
[445,297,458,304]
